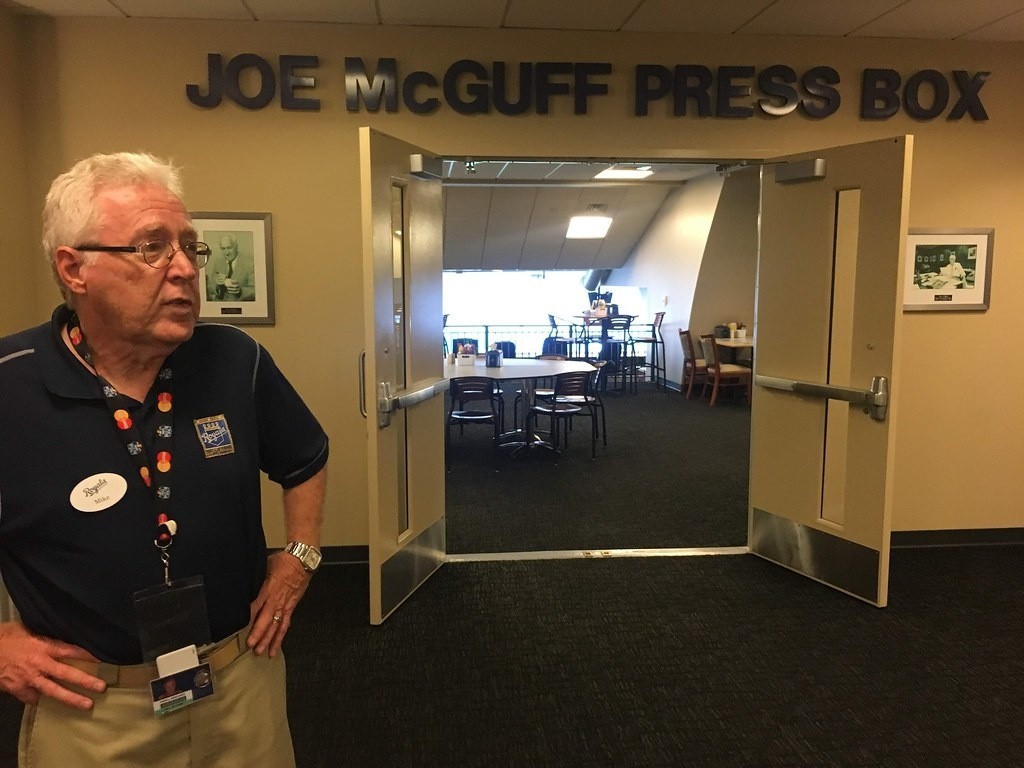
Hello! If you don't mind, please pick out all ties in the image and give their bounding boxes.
[226,262,233,279]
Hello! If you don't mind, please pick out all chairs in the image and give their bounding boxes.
[678,328,751,408]
[446,353,607,461]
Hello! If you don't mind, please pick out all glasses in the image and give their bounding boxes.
[75,240,212,270]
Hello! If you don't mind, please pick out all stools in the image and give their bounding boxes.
[443,314,450,358]
[548,312,666,394]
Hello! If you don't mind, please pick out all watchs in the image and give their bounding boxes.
[284,541,323,573]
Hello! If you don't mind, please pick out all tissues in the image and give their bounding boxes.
[486,343,503,368]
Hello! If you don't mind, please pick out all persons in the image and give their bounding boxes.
[0,151,331,768]
[208,234,254,301]
[940,255,966,277]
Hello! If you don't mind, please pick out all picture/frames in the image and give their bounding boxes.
[186,211,275,325]
[903,227,995,311]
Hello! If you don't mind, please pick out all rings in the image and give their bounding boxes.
[274,616,282,623]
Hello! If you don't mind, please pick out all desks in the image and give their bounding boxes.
[574,315,639,396]
[443,358,597,456]
[703,338,753,401]
